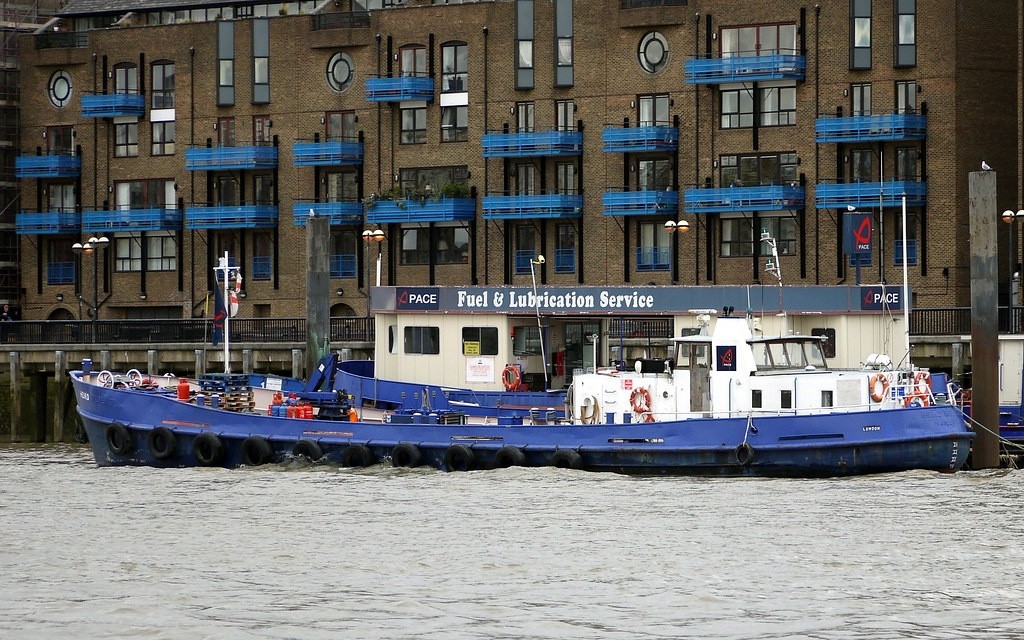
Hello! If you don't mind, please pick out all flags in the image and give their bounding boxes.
[212,276,227,346]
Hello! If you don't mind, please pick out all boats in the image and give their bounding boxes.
[67,232,977,478]
[933,365,1024,467]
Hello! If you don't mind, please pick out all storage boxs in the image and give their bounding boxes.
[497,416,523,425]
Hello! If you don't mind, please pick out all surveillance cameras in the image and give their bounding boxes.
[537,255,546,264]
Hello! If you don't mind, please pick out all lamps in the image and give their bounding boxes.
[336,288,344,296]
[56,294,63,301]
[723,306,735,318]
[140,293,147,299]
[240,290,247,298]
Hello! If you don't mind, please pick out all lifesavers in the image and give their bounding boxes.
[192,432,224,466]
[292,440,323,461]
[502,366,521,389]
[914,372,931,400]
[734,443,754,467]
[494,446,526,468]
[549,449,584,470]
[445,444,476,472]
[869,373,889,402]
[105,422,131,456]
[392,443,423,468]
[148,426,177,459]
[241,435,273,466]
[342,444,373,468]
[630,388,651,413]
[905,392,928,407]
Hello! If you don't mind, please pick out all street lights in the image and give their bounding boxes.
[1002,208,1024,335]
[71,235,109,342]
[663,219,689,285]
[363,229,386,342]
